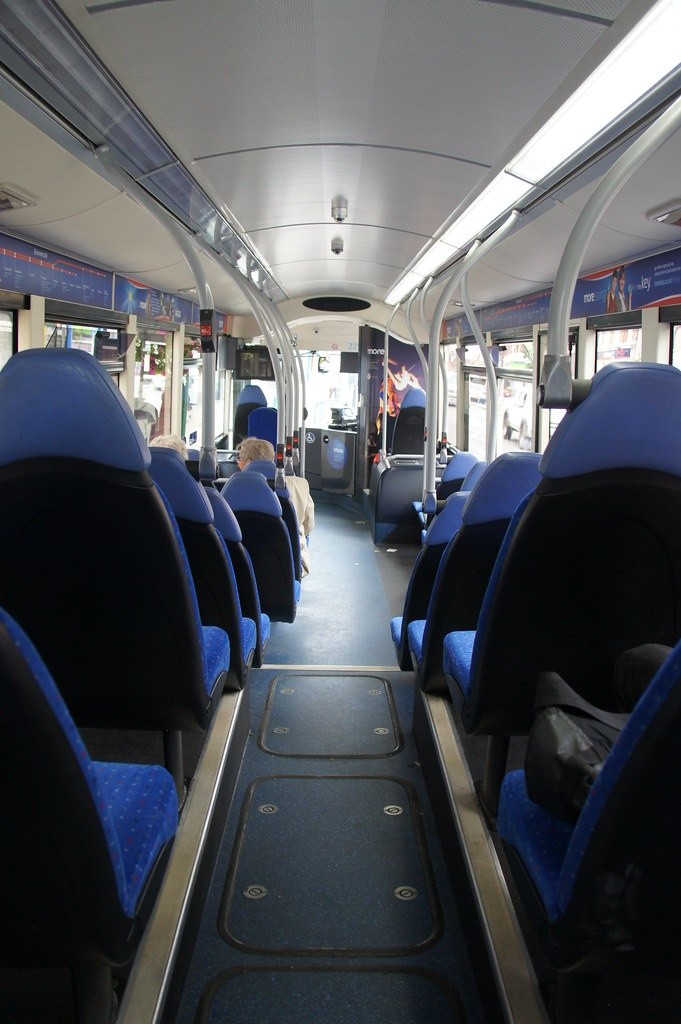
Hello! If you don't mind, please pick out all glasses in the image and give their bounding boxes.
[236,457,248,464]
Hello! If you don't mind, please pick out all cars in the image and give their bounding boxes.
[502,381,568,455]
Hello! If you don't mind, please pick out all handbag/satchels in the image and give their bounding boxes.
[525,671,634,828]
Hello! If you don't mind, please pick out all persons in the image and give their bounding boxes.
[236,436,315,578]
[150,434,189,466]
[526,642,674,815]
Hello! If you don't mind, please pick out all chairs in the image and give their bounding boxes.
[405,452,544,691]
[220,471,300,624]
[390,461,491,671]
[0,348,231,735]
[418,451,480,529]
[232,385,267,449]
[441,361,681,734]
[243,460,303,584]
[391,388,426,456]
[147,446,257,692]
[497,638,681,930]
[0,606,179,930]
[421,462,488,547]
[203,486,272,668]
